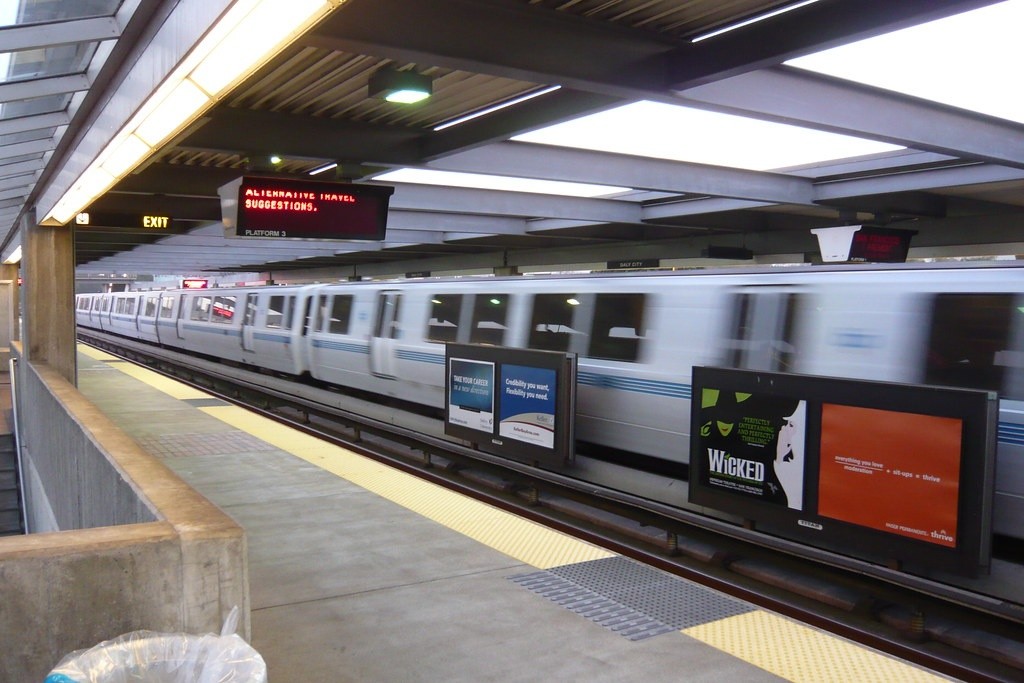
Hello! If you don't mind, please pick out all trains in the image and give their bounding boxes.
[70,259,1023,551]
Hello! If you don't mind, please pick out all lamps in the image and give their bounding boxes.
[367,66,433,105]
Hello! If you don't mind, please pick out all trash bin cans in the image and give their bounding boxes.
[43,631,268,683]
